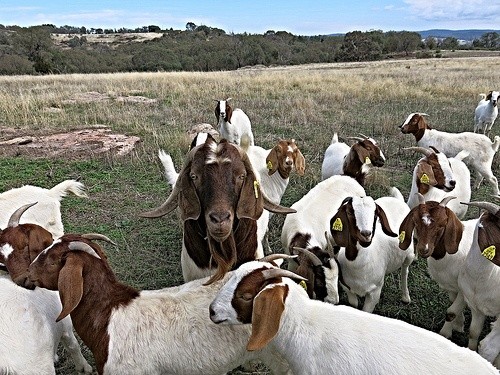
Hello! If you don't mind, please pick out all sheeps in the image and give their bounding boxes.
[0,89,500,375]
